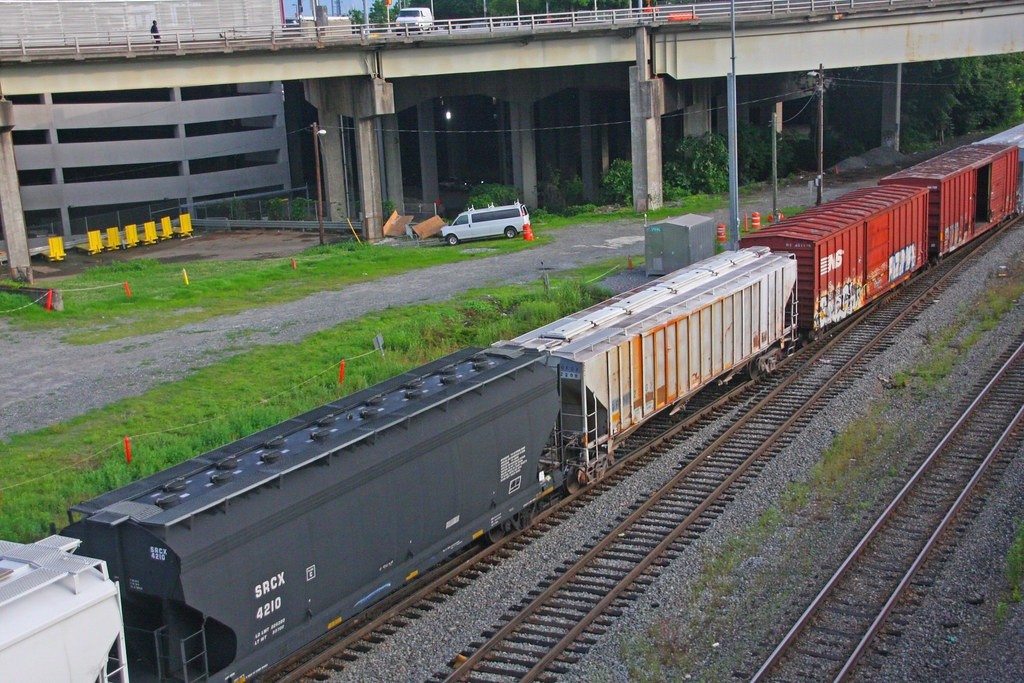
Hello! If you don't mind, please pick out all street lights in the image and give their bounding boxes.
[807,71,824,205]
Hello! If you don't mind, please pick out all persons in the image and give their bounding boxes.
[151,20,161,50]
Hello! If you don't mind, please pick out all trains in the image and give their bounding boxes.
[0,122,1023,683]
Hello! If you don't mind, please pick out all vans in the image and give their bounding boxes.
[396,6,433,35]
[438,202,531,244]
